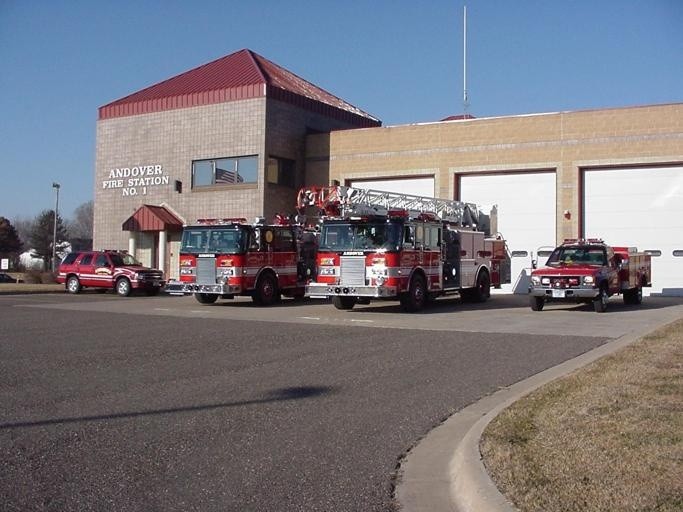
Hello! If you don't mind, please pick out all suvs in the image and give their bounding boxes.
[55,246,169,299]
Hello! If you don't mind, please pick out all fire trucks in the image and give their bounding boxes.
[291,186,511,314]
[161,213,366,310]
[522,235,654,311]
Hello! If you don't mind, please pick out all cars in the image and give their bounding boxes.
[0,274,23,284]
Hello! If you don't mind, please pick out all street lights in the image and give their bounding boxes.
[52,181,60,276]
